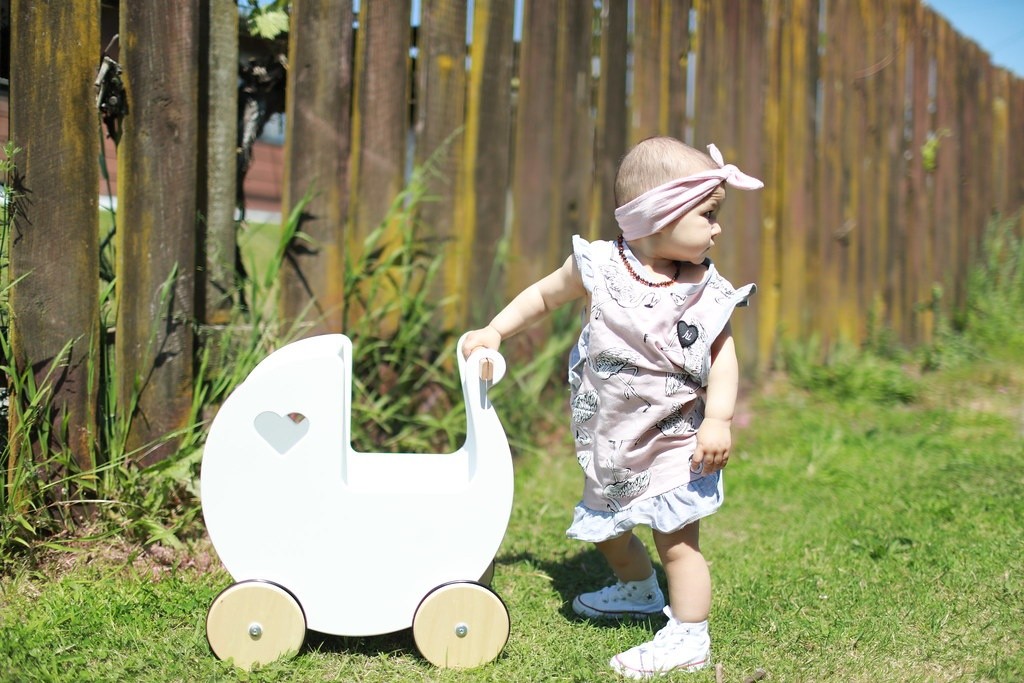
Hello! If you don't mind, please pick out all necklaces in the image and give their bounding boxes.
[617,234,681,288]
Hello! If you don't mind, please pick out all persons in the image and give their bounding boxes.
[461,136,765,680]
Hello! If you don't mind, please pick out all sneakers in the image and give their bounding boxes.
[609,605,711,680]
[573,568,665,619]
